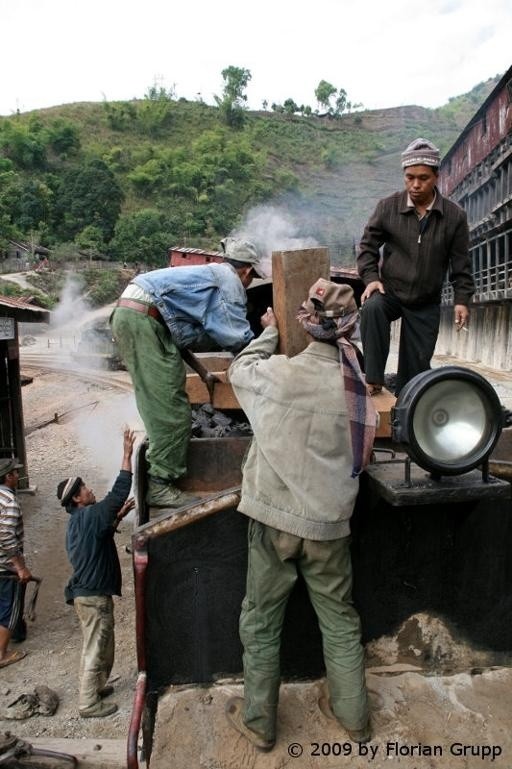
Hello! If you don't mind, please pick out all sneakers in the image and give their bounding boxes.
[101,681,115,698]
[331,701,376,744]
[224,696,275,754]
[80,701,117,719]
[144,477,202,509]
[362,384,382,393]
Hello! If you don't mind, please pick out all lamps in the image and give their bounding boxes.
[388,364,512,487]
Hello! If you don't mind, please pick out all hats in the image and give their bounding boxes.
[55,476,84,506]
[301,278,358,319]
[399,135,442,167]
[1,456,24,475]
[226,238,269,282]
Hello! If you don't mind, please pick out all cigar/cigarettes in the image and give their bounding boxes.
[456,324,470,333]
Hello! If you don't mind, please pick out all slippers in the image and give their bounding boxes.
[2,647,27,667]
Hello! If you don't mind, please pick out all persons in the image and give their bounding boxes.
[229,277,386,752]
[110,236,257,509]
[355,135,476,398]
[57,428,137,718]
[0,457,31,668]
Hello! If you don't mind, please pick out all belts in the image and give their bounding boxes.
[113,295,161,319]
